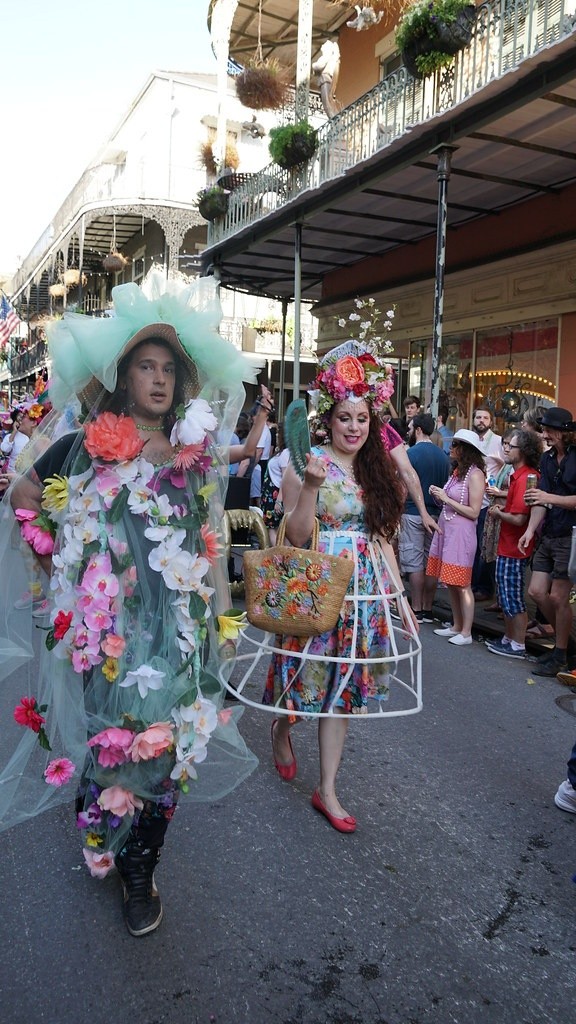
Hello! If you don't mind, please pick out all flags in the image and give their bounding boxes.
[0,287,21,348]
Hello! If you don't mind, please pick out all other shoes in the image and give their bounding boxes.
[484,601,502,612]
[448,634,473,645]
[484,618,576,693]
[471,586,478,592]
[473,591,490,601]
[223,681,242,701]
[433,627,461,637]
[554,778,576,814]
[496,613,504,619]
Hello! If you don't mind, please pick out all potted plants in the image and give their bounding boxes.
[191,186,229,220]
[394,1,476,78]
[268,118,318,169]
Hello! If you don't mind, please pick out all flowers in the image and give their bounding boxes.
[12,397,252,879]
[307,297,396,449]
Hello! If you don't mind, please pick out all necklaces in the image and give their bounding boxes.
[151,441,179,466]
[136,425,165,431]
[327,443,355,481]
[23,434,48,449]
[443,464,475,521]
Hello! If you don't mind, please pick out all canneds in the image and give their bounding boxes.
[525,474,537,502]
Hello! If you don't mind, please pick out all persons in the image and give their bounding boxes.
[218,339,422,834]
[554,742,576,813]
[0,367,60,618]
[485,407,576,687]
[311,39,340,120]
[0,276,258,936]
[386,393,504,645]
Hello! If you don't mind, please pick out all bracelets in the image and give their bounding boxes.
[402,589,406,596]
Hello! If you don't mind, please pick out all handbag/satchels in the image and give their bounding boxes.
[244,513,354,638]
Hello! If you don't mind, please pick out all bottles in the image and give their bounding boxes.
[488,475,496,487]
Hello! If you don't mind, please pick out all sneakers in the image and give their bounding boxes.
[421,610,433,623]
[114,844,163,935]
[75,775,91,819]
[390,606,423,624]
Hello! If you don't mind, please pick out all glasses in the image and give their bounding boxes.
[509,444,521,449]
[503,441,509,446]
[452,441,462,448]
[20,414,30,419]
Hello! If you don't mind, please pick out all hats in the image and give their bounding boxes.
[444,429,486,456]
[76,322,202,425]
[536,407,576,431]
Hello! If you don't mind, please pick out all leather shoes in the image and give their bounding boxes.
[271,719,297,780]
[312,791,356,833]
